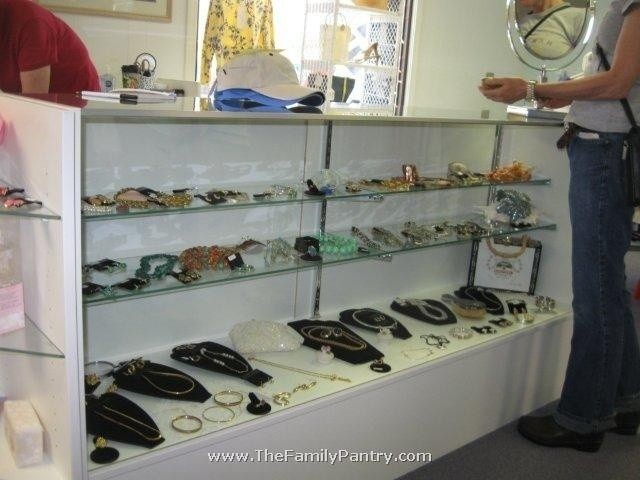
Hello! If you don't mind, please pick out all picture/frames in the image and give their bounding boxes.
[46,0,173,22]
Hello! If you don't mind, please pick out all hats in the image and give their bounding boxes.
[214,49,325,108]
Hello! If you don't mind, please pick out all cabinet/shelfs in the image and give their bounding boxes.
[301,0,414,108]
[0,91,572,480]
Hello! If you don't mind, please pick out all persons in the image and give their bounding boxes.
[0,0,101,93]
[478,0,640,453]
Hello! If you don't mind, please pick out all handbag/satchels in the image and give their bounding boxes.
[624,126,639,208]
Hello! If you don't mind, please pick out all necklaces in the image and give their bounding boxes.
[92,286,502,440]
[79,158,533,298]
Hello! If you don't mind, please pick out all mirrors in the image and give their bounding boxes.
[504,0,596,120]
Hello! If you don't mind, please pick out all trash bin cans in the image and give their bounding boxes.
[306,63,357,102]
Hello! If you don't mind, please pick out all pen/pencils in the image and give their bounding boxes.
[75,91,138,99]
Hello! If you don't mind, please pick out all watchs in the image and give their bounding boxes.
[525,79,538,102]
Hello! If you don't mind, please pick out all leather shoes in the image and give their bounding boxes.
[608,412,640,435]
[516,413,602,452]
